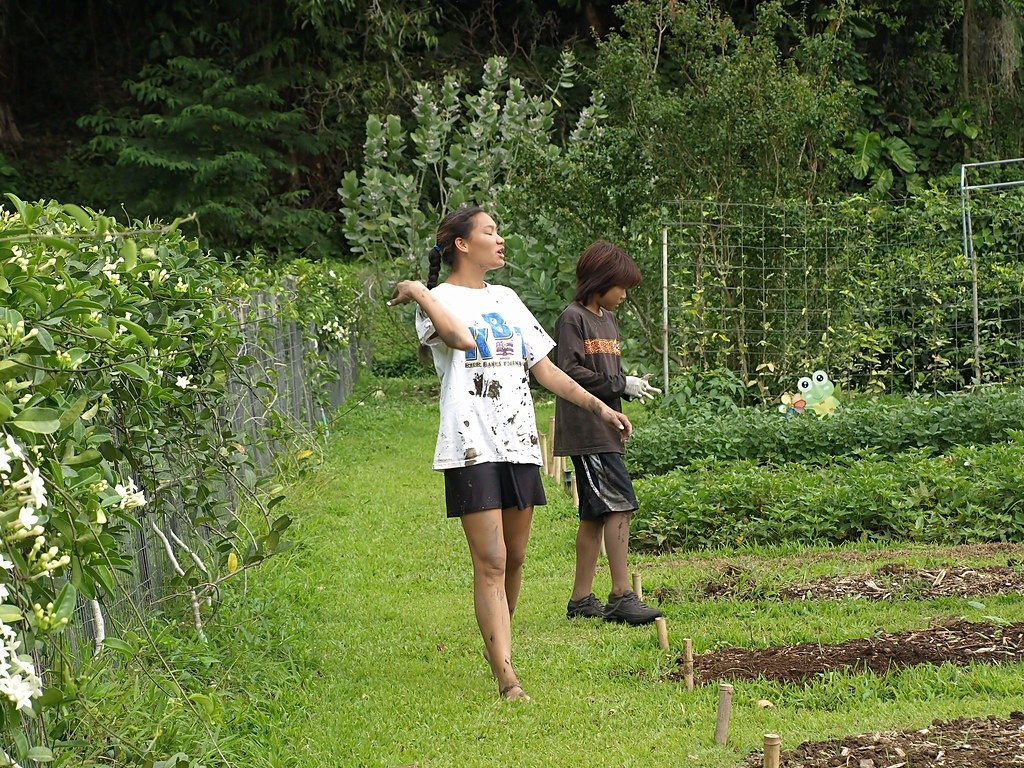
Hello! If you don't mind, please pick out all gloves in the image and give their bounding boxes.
[623,376,647,397]
[629,374,662,404]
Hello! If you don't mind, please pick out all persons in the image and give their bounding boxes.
[553,241,664,625]
[387,208,632,700]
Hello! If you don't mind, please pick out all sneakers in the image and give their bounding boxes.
[567,593,606,617]
[603,590,662,624]
